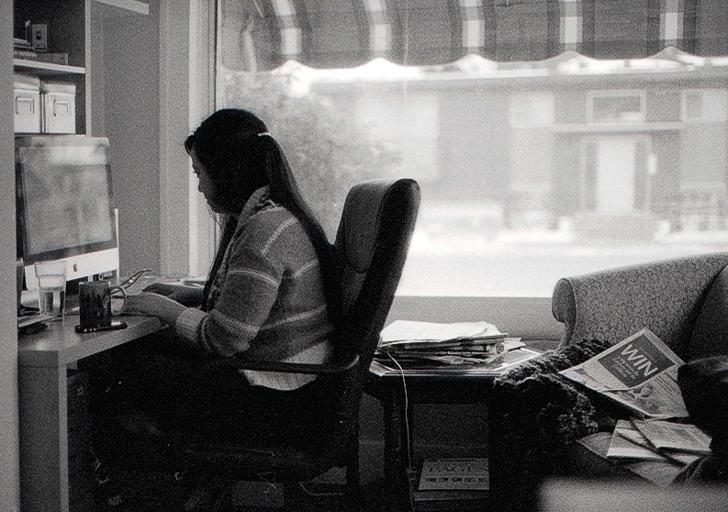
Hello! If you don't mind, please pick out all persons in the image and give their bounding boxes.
[87,107,331,471]
[630,383,654,400]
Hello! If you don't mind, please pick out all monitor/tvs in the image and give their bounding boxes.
[14,131,122,292]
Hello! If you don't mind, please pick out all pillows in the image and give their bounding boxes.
[491,336,637,475]
[670,356,727,487]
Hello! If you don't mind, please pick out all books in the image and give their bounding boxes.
[417,454,492,491]
[378,319,509,365]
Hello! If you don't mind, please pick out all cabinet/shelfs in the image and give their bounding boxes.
[13,1,94,140]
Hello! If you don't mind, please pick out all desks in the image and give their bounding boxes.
[19,297,169,512]
[363,342,546,511]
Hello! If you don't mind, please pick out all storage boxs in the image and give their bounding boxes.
[14,74,42,134]
[42,80,77,134]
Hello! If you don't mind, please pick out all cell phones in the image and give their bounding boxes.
[74,320,129,334]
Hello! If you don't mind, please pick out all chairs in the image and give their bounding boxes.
[491,249,728,510]
[160,177,420,512]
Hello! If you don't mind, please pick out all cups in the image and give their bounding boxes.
[35,259,67,328]
[78,280,127,326]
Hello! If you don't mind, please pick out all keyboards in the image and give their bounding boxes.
[111,299,147,317]
